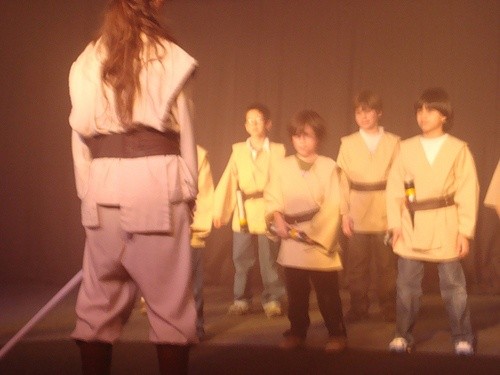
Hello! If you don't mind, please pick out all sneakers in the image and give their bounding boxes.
[388,336,410,354]
[454,340,473,356]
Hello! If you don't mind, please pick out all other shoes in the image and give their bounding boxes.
[279,334,301,349]
[228,298,254,315]
[344,306,366,324]
[323,339,348,353]
[261,299,283,319]
[384,307,396,321]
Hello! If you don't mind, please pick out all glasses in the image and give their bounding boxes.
[245,116,265,125]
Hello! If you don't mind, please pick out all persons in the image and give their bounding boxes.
[190,143,213,337]
[385,87,480,356]
[336,89,405,320]
[484,163,500,220]
[70,0,199,375]
[263,110,347,353]
[213,102,287,317]
